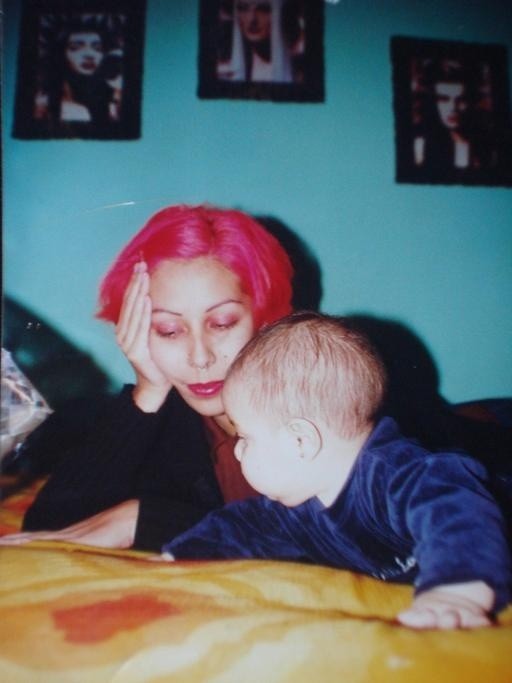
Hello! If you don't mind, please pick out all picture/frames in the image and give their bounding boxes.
[194,0,328,103]
[10,0,146,142]
[389,34,511,188]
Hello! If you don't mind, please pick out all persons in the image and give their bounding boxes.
[214,1,296,82]
[0,198,294,551]
[35,24,119,120]
[142,307,511,632]
[413,67,474,167]
